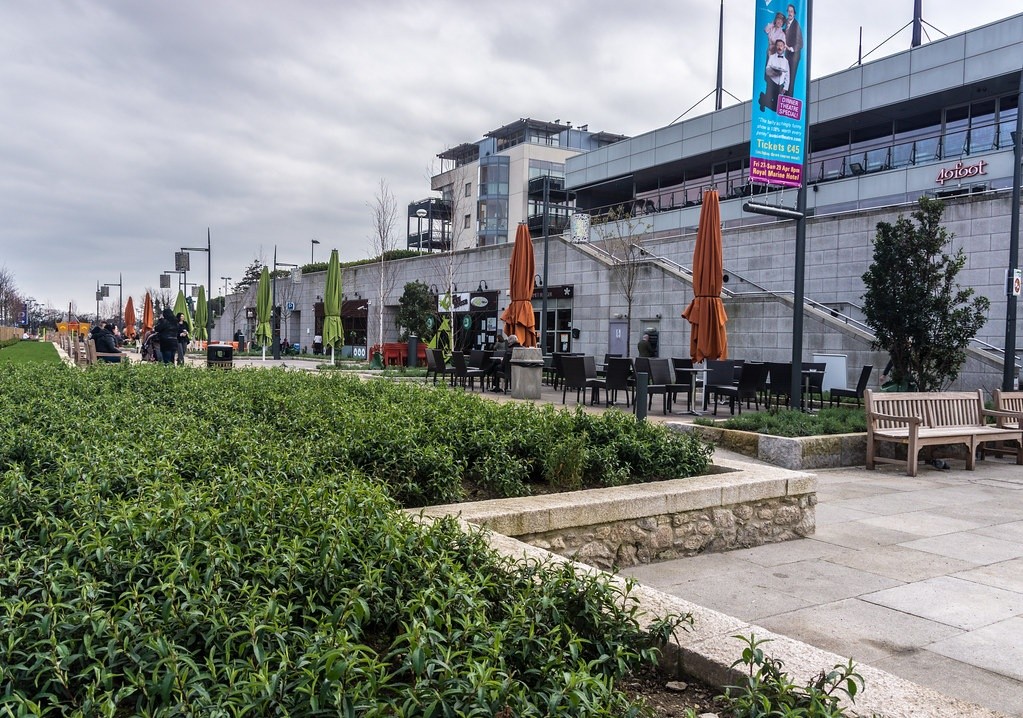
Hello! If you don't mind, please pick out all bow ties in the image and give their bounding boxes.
[777,55,783,58]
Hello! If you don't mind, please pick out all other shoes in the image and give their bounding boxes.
[490,386,500,392]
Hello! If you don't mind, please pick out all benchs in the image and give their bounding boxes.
[864,388,1023,477]
[993,388,1023,465]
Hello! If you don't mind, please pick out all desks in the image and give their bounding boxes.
[675,365,742,417]
[593,362,634,372]
[447,354,503,364]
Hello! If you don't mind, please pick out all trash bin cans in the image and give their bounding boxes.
[207,345,234,370]
[509,347,545,399]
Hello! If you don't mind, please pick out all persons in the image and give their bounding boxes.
[314,330,322,355]
[758,4,803,112]
[637,334,655,357]
[23,331,30,340]
[91,321,139,363]
[155,309,178,364]
[176,313,190,366]
[484,335,523,392]
[234,329,289,351]
[494,335,505,350]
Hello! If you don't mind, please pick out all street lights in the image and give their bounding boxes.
[101,284,121,332]
[160,271,186,299]
[272,263,302,338]
[175,248,211,345]
[96,291,103,327]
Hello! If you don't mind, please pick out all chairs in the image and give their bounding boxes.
[186,340,250,353]
[830,365,873,410]
[134,339,143,354]
[59,332,127,365]
[704,360,827,416]
[425,347,512,394]
[542,352,698,417]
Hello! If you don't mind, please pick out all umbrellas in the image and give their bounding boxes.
[254,265,272,362]
[123,297,136,339]
[323,248,344,365]
[195,285,208,351]
[500,220,537,348]
[681,166,727,412]
[142,293,154,335]
[173,290,192,340]
